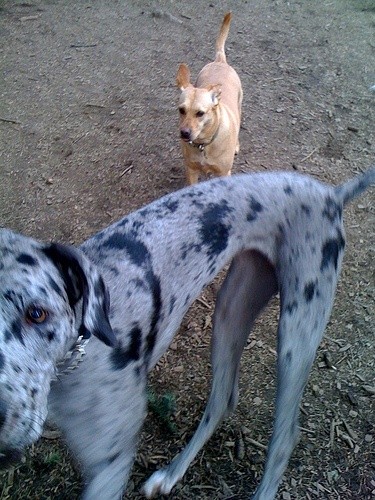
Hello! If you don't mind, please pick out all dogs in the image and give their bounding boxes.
[0,166,375,499]
[177,13,244,186]
